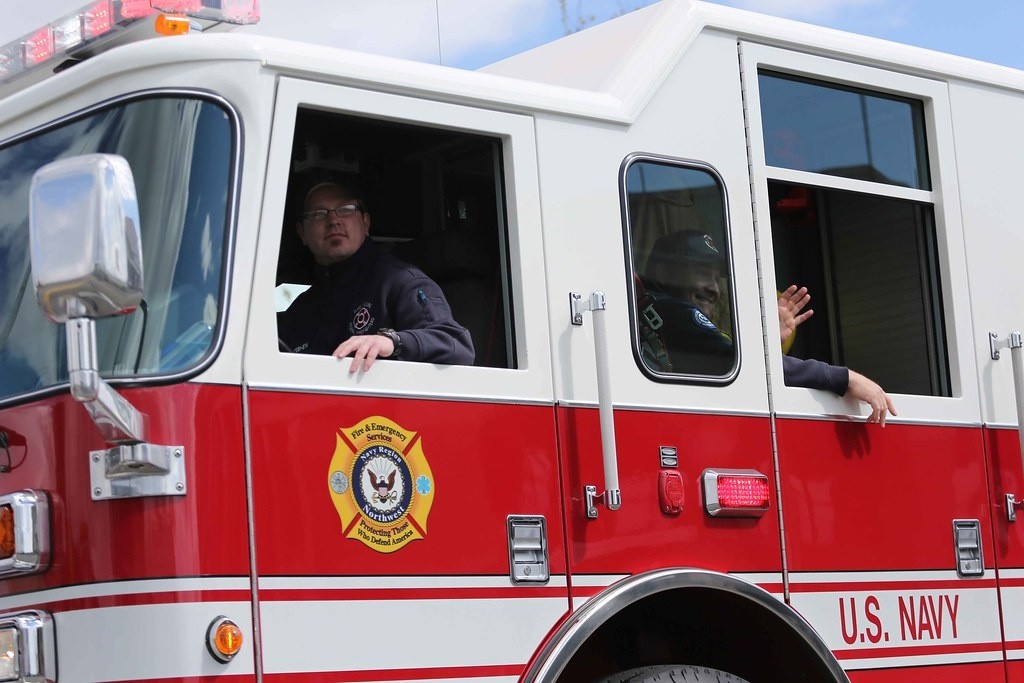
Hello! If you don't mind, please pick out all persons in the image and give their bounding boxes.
[643,230,898,428]
[278,174,476,373]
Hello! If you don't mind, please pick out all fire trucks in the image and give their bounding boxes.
[2,0,1024,683]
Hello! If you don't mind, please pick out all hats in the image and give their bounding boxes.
[297,164,354,218]
[646,229,726,265]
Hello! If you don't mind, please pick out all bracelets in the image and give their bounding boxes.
[377,328,403,360]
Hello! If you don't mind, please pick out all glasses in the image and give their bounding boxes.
[300,204,362,220]
